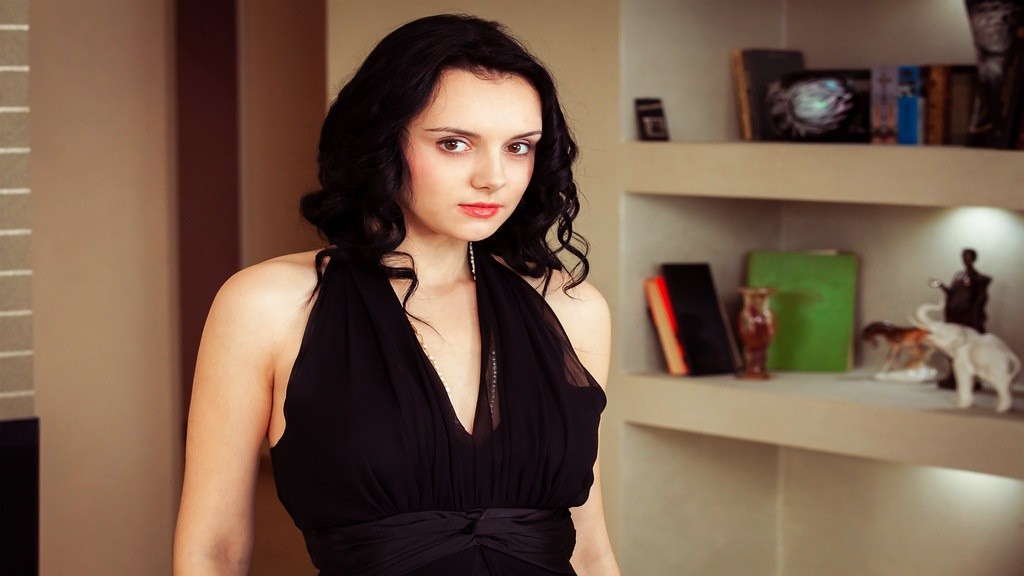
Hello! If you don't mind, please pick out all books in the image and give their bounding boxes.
[747,247,857,371]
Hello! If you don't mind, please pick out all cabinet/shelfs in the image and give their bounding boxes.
[620,139,1024,482]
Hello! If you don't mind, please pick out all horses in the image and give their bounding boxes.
[861,320,937,374]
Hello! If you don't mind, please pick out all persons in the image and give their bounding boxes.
[172,12,621,576]
[927,249,991,390]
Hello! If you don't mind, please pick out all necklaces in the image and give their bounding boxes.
[407,241,498,424]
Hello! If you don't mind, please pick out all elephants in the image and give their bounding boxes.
[908,296,1021,413]
